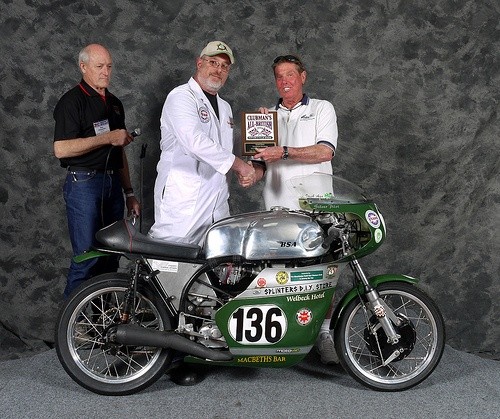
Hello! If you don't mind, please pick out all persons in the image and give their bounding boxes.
[239,55,342,368]
[148,42,255,386]
[52,44,140,350]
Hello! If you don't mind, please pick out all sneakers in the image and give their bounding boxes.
[316,332,339,365]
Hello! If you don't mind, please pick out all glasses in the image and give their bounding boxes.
[201,57,230,71]
[274,55,304,71]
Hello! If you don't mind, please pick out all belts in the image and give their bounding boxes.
[69,167,113,174]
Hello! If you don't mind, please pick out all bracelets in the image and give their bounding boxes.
[123,188,135,196]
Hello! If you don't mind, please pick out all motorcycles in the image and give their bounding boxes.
[50,170,446,396]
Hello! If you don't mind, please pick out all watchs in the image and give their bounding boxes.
[281,146,289,160]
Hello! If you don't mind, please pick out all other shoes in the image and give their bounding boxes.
[73,319,92,349]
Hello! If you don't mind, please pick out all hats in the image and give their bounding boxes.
[200,41,234,65]
